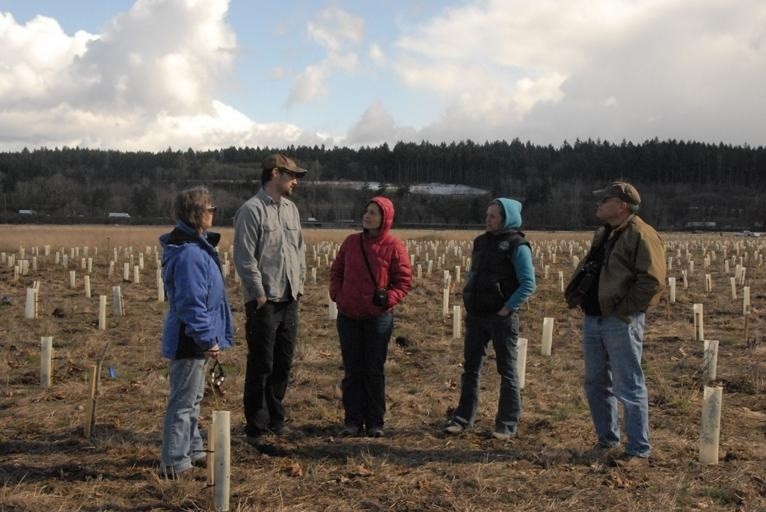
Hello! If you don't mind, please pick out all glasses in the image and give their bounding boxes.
[205,206,218,213]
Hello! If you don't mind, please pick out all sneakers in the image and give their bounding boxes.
[247,422,305,456]
[342,422,385,438]
[608,450,649,468]
[158,453,208,481]
[491,429,517,440]
[442,421,464,434]
[584,440,622,458]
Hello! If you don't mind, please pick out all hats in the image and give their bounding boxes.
[262,154,308,179]
[592,181,642,207]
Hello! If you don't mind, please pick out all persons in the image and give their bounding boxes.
[234,154,309,445]
[444,198,537,443]
[564,181,666,467]
[158,187,236,483]
[329,196,413,438]
